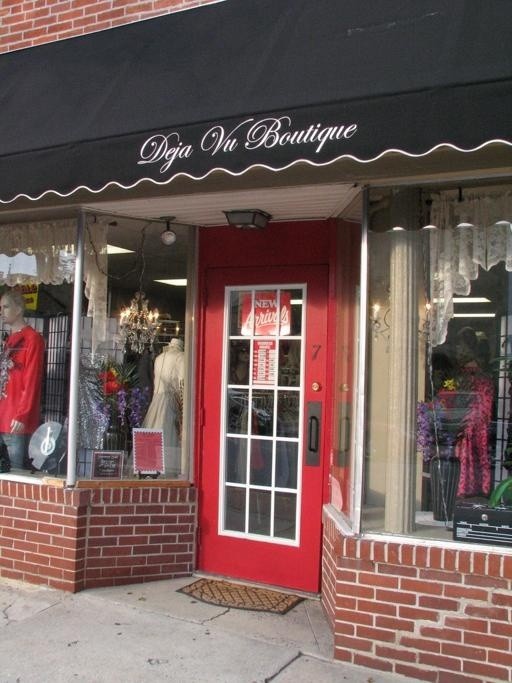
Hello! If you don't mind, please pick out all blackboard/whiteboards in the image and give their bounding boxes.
[90,451,123,480]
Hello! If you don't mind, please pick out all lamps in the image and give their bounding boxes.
[221,209,272,232]
[368,303,391,353]
[158,216,178,246]
[115,220,162,355]
[418,302,434,343]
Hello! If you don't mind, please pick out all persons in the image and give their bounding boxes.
[0,287,48,468]
[229,345,264,475]
[450,327,494,499]
[124,335,184,481]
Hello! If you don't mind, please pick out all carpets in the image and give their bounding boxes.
[175,578,305,614]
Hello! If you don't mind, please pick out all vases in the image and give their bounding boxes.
[437,391,474,408]
[429,445,460,522]
[103,409,126,462]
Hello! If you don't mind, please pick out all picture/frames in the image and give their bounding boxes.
[132,427,167,476]
[91,450,123,480]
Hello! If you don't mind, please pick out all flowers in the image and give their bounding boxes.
[93,357,151,430]
[433,364,481,392]
[415,401,458,464]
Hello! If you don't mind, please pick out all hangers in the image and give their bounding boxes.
[231,394,300,429]
